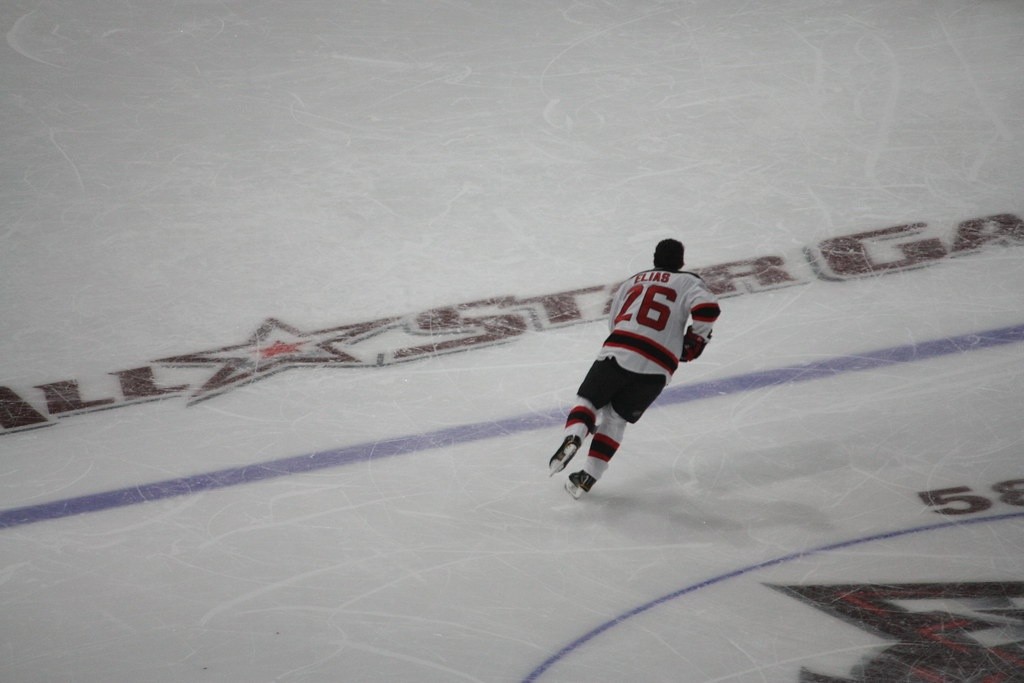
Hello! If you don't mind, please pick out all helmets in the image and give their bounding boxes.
[654,239,685,269]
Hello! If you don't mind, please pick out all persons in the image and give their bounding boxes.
[548,238,722,500]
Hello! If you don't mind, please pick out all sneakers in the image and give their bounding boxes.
[548,434,582,477]
[564,469,597,500]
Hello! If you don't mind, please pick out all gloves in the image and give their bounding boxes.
[679,324,712,363]
